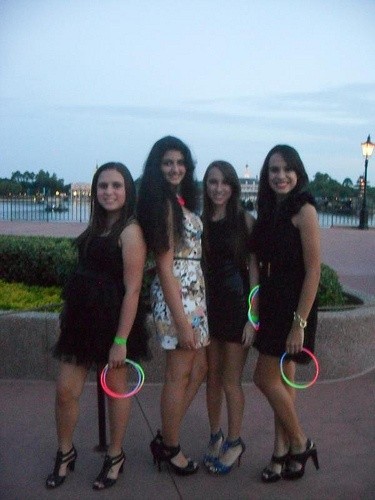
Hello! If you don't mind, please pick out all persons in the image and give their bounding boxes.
[199,161,259,475]
[255,145,322,482]
[137,135,211,473]
[47,162,146,490]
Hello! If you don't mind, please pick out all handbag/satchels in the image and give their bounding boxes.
[61,264,122,326]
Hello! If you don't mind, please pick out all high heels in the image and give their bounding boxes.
[207,436,246,476]
[261,448,291,483]
[46,443,77,489]
[203,428,224,472]
[282,438,319,480]
[92,448,126,490]
[150,431,180,464]
[155,439,200,475]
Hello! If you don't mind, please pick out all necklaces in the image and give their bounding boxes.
[178,198,185,205]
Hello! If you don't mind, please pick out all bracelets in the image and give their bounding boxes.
[293,312,307,328]
[251,316,258,321]
[114,337,127,345]
